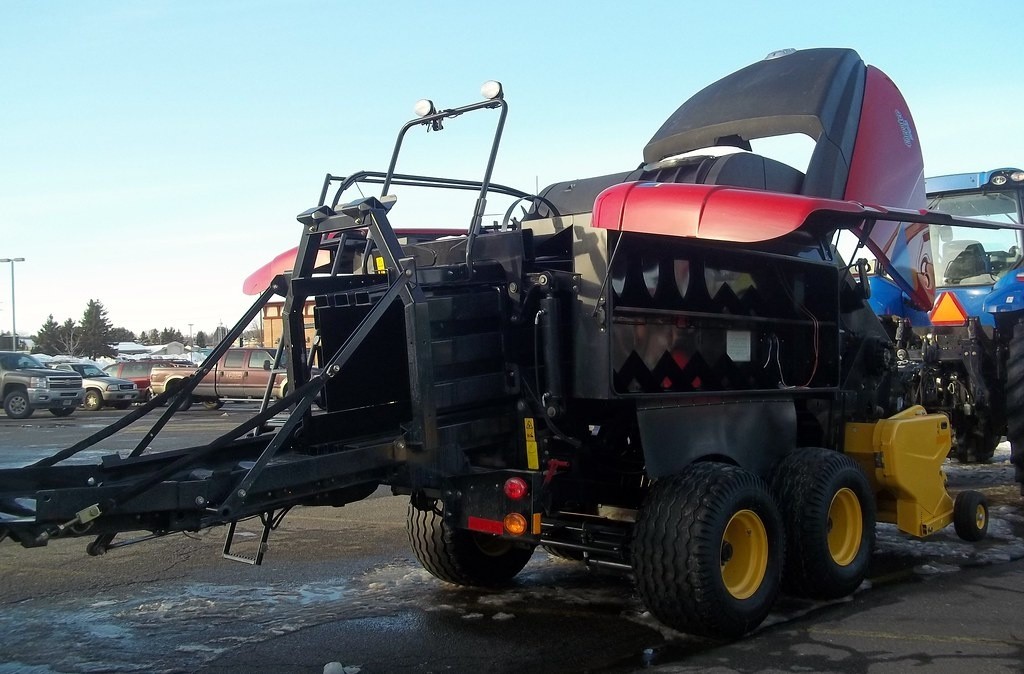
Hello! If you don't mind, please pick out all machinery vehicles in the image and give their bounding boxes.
[865,167,1024,496]
[0,48,989,635]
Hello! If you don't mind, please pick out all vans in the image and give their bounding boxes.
[95,358,198,395]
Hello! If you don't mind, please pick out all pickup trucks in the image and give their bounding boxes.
[148,348,323,411]
[51,363,140,411]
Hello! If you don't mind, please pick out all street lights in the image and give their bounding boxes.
[0,258,25,351]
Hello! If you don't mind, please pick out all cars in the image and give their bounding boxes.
[0,351,86,418]
